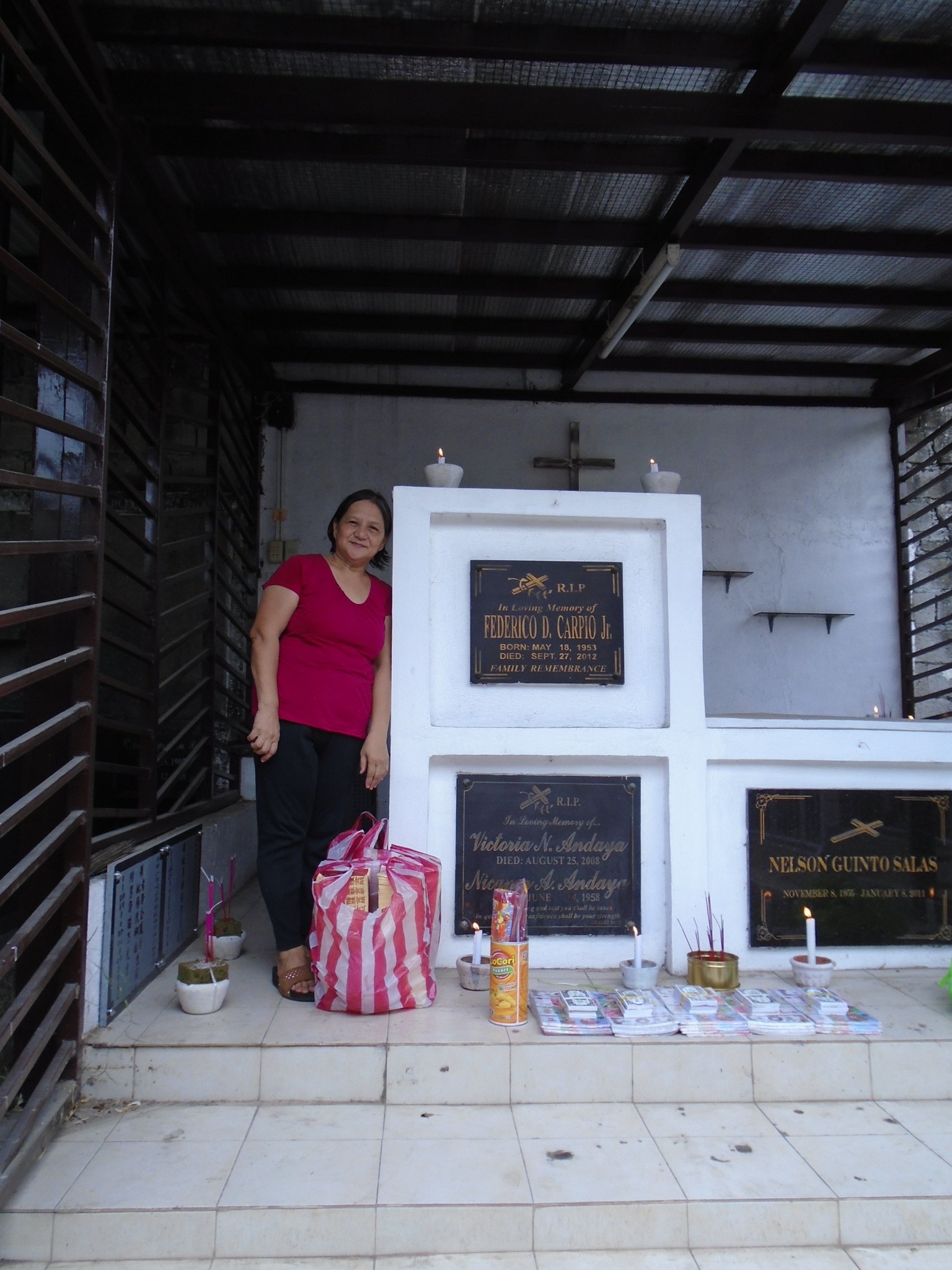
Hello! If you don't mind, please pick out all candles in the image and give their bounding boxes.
[874,706,879,718]
[633,926,642,968]
[650,459,658,472]
[438,448,445,464]
[473,923,482,964]
[804,906,816,965]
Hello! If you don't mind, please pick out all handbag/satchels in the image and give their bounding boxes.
[308,810,445,1013]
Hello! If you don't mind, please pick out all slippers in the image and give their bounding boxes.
[272,964,314,1002]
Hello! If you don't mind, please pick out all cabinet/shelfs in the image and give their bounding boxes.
[702,569,855,633]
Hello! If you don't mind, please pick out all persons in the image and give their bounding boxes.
[246,490,393,1002]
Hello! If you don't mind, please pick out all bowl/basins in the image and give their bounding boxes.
[456,955,490,991]
[424,463,464,486]
[790,956,833,989]
[687,952,740,991]
[204,931,248,961]
[620,959,659,990]
[176,979,230,1014]
[640,472,681,492]
